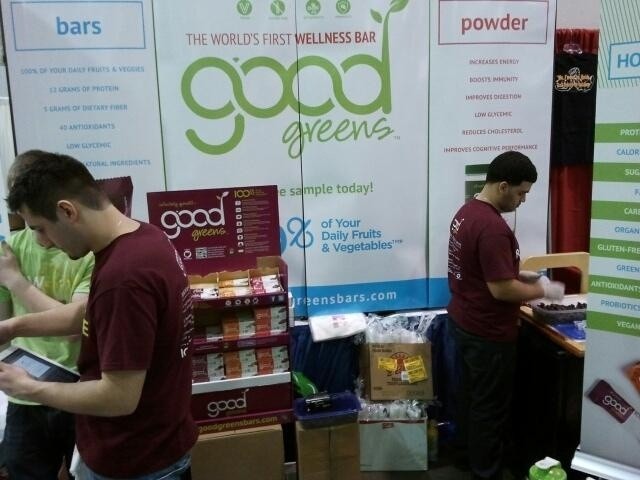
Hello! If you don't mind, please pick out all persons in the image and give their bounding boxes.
[0,152,201,480]
[446,149,567,479]
[1,150,97,480]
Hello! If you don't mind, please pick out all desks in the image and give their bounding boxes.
[515,294,587,479]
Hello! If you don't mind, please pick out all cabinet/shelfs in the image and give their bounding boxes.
[186,256,291,396]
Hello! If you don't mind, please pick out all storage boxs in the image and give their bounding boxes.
[190,423,286,480]
[293,339,434,479]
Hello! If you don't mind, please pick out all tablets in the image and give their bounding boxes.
[0,346,82,384]
